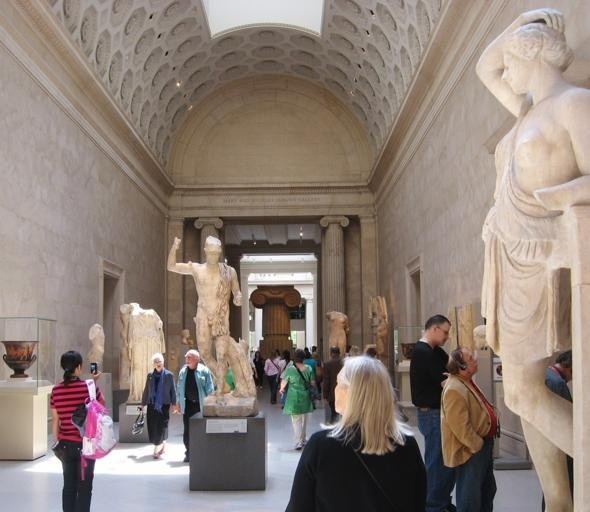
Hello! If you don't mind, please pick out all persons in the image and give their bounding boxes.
[473,5,590,512]
[409,314,458,512]
[440,345,501,511]
[472,326,489,352]
[253,346,324,454]
[179,328,190,346]
[324,345,344,425]
[165,233,244,407]
[175,349,215,464]
[137,353,177,460]
[49,350,107,512]
[541,349,573,512]
[284,350,427,511]
[324,309,349,358]
[126,302,167,408]
[366,342,377,359]
[349,343,359,354]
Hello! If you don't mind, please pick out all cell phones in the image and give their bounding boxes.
[90,363,97,375]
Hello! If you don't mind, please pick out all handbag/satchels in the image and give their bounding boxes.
[305,381,318,401]
[130,421,145,435]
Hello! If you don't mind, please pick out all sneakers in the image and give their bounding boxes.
[184,450,189,462]
[153,442,164,459]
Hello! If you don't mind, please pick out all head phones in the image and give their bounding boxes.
[450,352,468,370]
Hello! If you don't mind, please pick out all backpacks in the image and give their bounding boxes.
[71,378,117,460]
